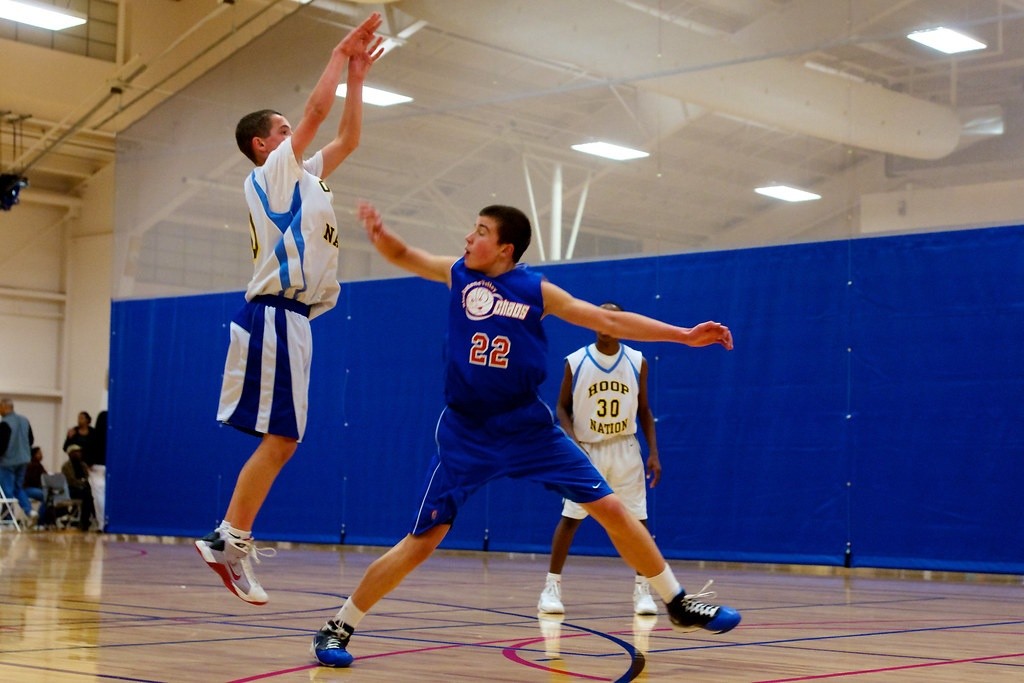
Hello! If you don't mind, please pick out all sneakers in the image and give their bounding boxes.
[538,615,563,629]
[632,582,657,614]
[194,528,276,605]
[634,616,656,629]
[310,620,354,667]
[666,580,741,635]
[538,589,564,614]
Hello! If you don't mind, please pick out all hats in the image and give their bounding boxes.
[67,444,81,453]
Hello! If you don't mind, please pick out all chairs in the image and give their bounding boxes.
[0,484,21,532]
[40,472,83,529]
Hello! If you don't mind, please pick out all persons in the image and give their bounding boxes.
[196,12,385,604]
[0,398,53,529]
[62,411,108,533]
[63,412,95,453]
[538,303,662,614]
[311,204,741,666]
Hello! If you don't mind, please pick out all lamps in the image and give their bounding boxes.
[0,0,91,31]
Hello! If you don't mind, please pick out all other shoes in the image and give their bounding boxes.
[26,510,38,528]
[80,525,88,531]
